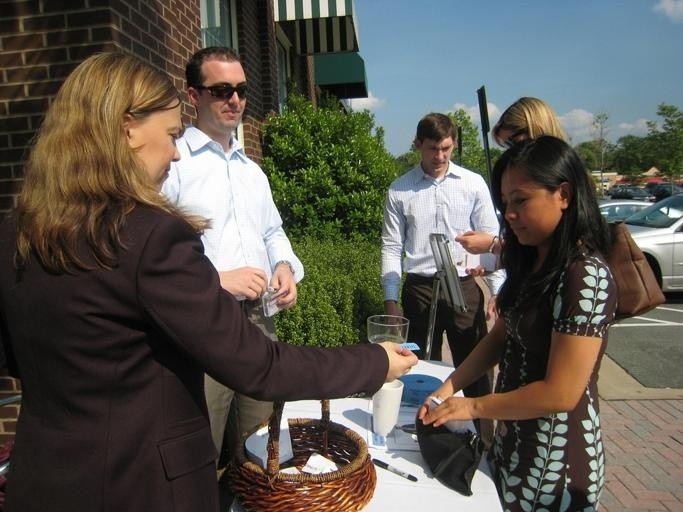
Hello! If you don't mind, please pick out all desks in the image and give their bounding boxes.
[230,359,502,512]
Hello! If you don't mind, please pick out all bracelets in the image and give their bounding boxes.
[489,236,499,254]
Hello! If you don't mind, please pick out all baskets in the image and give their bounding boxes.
[226,397,377,511]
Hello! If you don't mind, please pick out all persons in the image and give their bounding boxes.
[381,113,501,465]
[455,97,568,277]
[417,135,616,511]
[0,50,417,512]
[159,48,304,472]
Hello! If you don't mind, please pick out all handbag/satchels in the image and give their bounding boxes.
[413,411,484,495]
[600,221,665,319]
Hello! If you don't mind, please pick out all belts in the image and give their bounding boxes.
[239,298,263,310]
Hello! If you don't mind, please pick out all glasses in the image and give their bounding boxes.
[198,79,250,102]
[499,127,528,150]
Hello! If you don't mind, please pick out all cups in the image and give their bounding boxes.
[367,314,410,351]
[371,378,406,435]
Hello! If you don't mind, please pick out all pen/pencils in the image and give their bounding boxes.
[372,458,418,482]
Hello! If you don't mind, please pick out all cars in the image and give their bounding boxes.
[608,184,657,203]
[646,182,683,201]
[598,199,682,227]
[621,194,682,294]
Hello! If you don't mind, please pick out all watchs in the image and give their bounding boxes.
[273,259,295,275]
